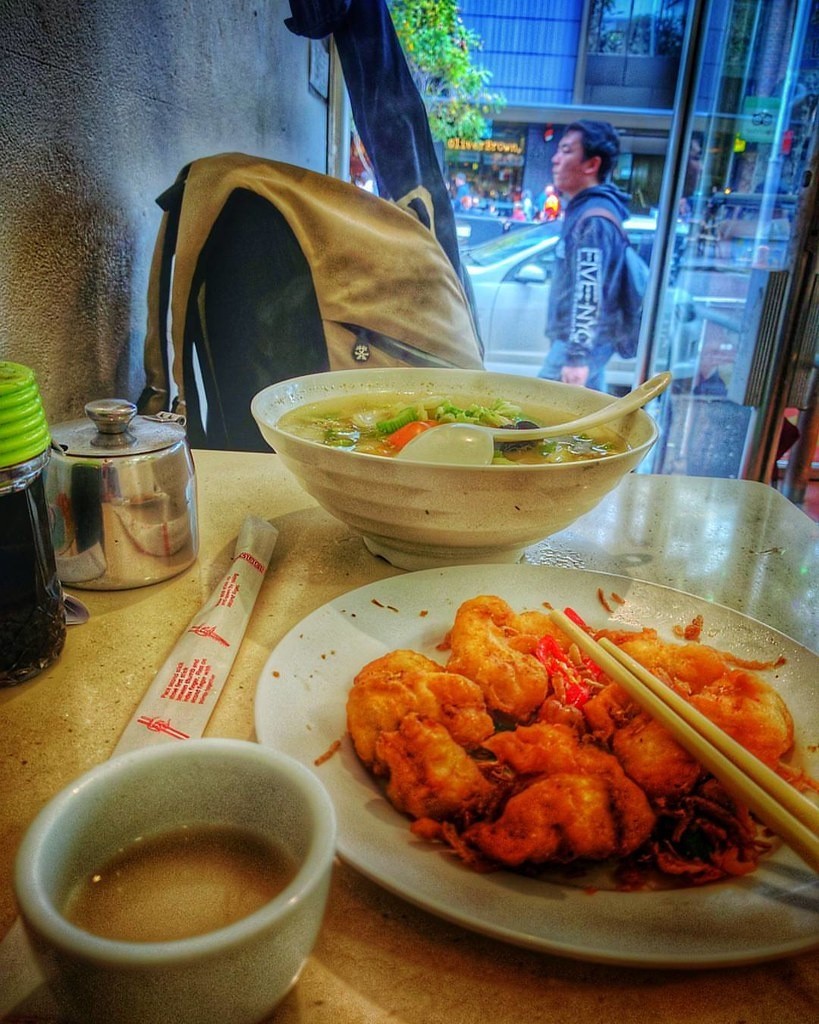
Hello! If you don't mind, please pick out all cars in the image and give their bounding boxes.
[462,214,697,388]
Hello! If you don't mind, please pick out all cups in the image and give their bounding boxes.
[15,737,336,1024]
[43,399,199,592]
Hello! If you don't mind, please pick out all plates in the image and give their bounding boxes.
[253,562,819,963]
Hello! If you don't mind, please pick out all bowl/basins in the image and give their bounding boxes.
[252,368,660,571]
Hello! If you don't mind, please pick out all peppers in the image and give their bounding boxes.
[537,606,612,709]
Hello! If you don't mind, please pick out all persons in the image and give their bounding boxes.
[537,118,633,394]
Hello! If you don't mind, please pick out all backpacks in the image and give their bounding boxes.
[571,210,654,360]
[134,150,486,454]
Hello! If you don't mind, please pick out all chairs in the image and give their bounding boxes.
[139,157,484,452]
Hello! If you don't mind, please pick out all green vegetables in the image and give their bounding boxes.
[325,399,616,457]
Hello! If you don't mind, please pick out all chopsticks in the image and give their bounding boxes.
[548,607,819,870]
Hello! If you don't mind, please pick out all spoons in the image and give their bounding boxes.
[397,371,671,466]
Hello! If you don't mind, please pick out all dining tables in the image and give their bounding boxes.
[0,449,819,1024]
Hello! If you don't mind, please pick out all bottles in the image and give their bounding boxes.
[0,361,66,688]
